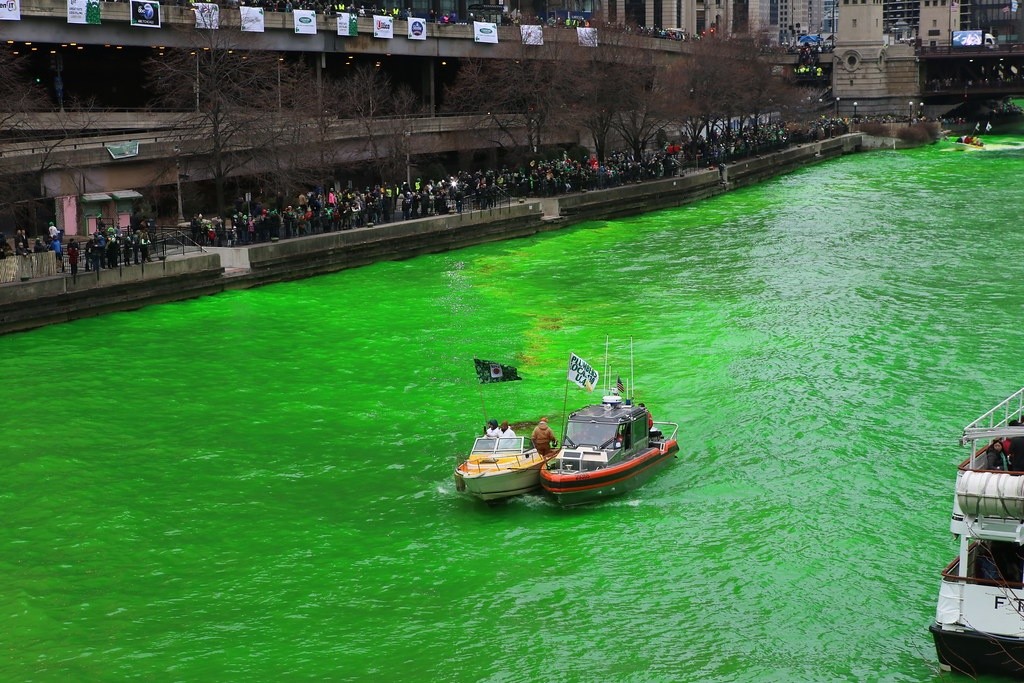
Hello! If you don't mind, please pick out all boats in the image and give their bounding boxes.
[927,386,1024,681]
[453,436,560,501]
[540,388,680,506]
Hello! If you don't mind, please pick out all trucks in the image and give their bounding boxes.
[952,29,998,49]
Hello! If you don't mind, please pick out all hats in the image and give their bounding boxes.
[541,418,547,422]
[49,222,55,226]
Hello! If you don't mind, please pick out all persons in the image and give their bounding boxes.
[986,415,1024,472]
[481,419,518,448]
[601,427,623,448]
[531,417,556,456]
[187,0,831,80]
[0,209,152,275]
[638,403,654,442]
[190,103,1024,247]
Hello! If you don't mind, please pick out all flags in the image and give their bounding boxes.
[986,122,992,131]
[975,122,980,131]
[566,352,599,393]
[1002,6,1009,13]
[474,358,522,384]
[617,376,625,393]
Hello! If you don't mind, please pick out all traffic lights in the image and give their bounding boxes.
[710,29,714,33]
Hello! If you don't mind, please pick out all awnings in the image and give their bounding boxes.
[80,192,112,203]
[111,190,143,200]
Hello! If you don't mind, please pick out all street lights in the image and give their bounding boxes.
[191,52,199,113]
[404,130,412,188]
[174,145,185,223]
[278,57,284,119]
